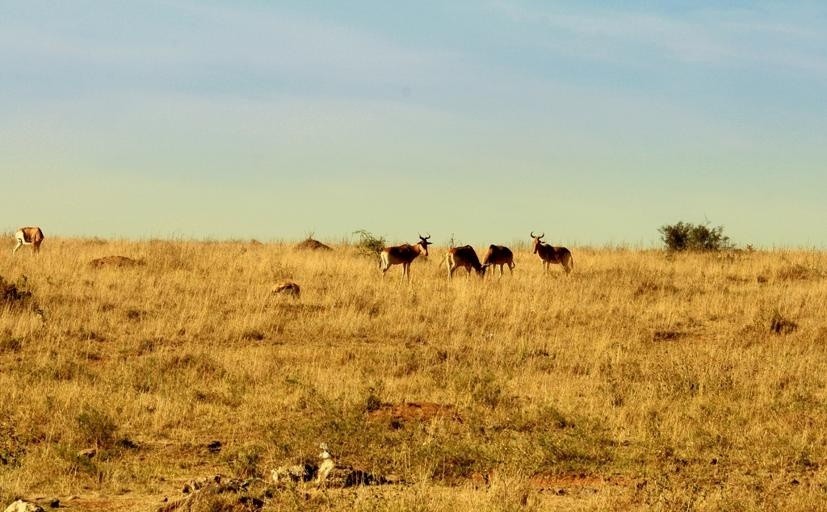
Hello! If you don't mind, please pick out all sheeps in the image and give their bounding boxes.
[529,231,574,275]
[446,242,516,277]
[376,232,432,279]
[13,225,45,255]
